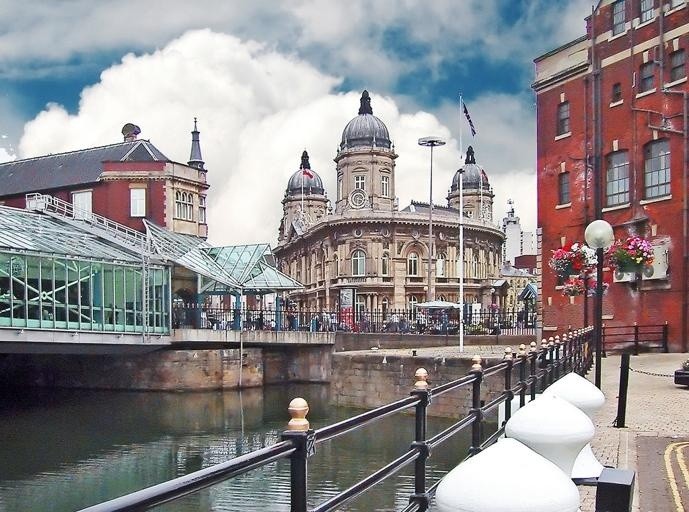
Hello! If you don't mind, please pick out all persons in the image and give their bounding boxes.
[1,290,504,336]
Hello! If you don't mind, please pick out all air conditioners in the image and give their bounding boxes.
[613,270,635,284]
[641,265,668,281]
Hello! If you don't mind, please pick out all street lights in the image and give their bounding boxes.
[490,287,495,305]
[583,219,614,387]
[423,286,429,302]
